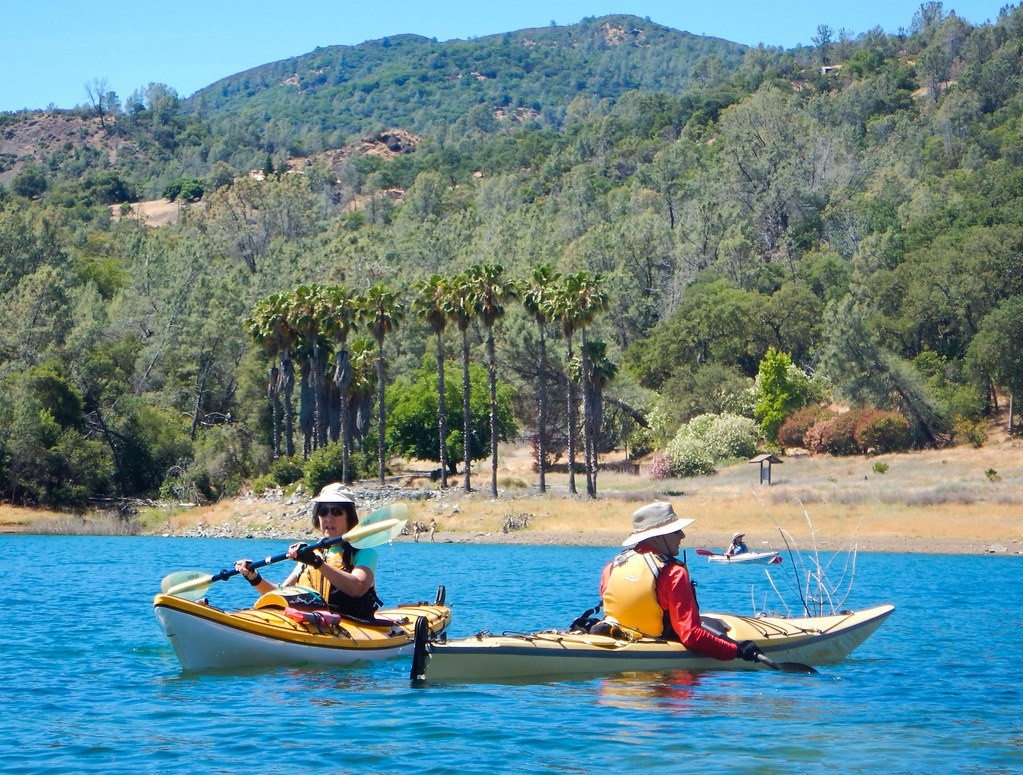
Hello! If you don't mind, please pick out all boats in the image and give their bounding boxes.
[409,602,894,687]
[153,588,452,670]
[707,549,780,566]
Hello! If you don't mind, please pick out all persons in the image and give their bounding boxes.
[600,502,762,661]
[234,483,384,626]
[429,517,437,543]
[414,521,420,543]
[726,533,748,557]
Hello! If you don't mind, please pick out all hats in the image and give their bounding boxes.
[733,533,745,540]
[309,483,355,502]
[622,502,695,546]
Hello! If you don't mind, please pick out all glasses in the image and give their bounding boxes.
[319,507,343,516]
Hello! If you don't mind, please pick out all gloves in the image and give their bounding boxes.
[736,640,763,661]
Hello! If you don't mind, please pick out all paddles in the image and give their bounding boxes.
[696,550,783,564]
[700,622,819,674]
[161,503,407,603]
